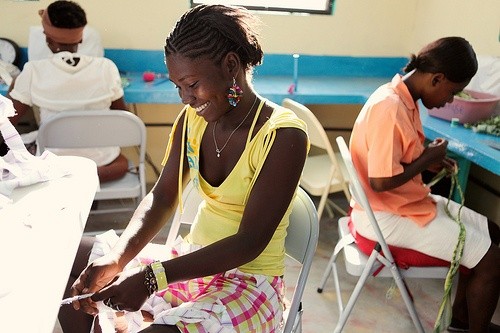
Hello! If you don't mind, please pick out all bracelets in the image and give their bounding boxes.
[151,260,169,294]
[143,265,158,297]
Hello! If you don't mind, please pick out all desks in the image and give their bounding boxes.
[0,155,102,333]
[118,70,500,206]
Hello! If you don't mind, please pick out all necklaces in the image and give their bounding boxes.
[212,95,257,157]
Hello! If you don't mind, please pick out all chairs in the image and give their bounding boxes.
[278,186,319,333]
[281,97,355,220]
[38,110,148,236]
[318,135,452,333]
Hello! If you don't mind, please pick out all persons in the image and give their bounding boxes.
[350,37,500,333]
[8,0,131,166]
[57,4,310,333]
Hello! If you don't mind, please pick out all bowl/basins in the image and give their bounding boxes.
[428,88,500,122]
